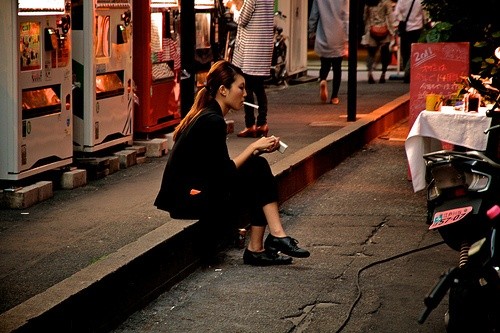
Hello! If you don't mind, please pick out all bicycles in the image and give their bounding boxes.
[226,11,288,87]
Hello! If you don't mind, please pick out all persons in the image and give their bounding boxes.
[154,59,311,267]
[308,0,349,104]
[360,0,430,83]
[230,0,275,138]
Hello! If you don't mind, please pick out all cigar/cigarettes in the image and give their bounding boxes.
[242,102,260,110]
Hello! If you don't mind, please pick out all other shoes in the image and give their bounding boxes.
[379,78,385,83]
[320,80,329,101]
[368,76,375,83]
[330,98,339,105]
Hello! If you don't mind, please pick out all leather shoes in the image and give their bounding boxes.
[264,233,312,258]
[243,246,293,265]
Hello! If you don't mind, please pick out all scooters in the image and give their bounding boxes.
[416,100,500,333]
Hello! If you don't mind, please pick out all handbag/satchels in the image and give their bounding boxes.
[370,24,388,39]
[398,20,406,35]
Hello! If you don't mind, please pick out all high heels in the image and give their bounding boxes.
[257,124,269,137]
[237,125,258,138]
